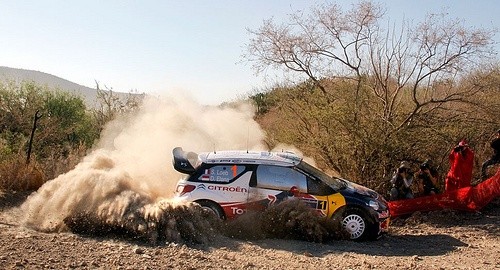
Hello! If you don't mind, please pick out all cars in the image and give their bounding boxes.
[171,147,390,243]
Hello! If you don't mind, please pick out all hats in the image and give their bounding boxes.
[458,140,471,147]
[424,159,435,168]
[399,160,410,170]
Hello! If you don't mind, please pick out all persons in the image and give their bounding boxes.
[445,140,474,191]
[480,129,500,180]
[415,159,441,197]
[390,161,415,200]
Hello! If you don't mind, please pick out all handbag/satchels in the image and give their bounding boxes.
[389,187,398,198]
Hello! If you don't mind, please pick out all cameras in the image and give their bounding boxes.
[454,145,464,152]
[399,167,406,173]
